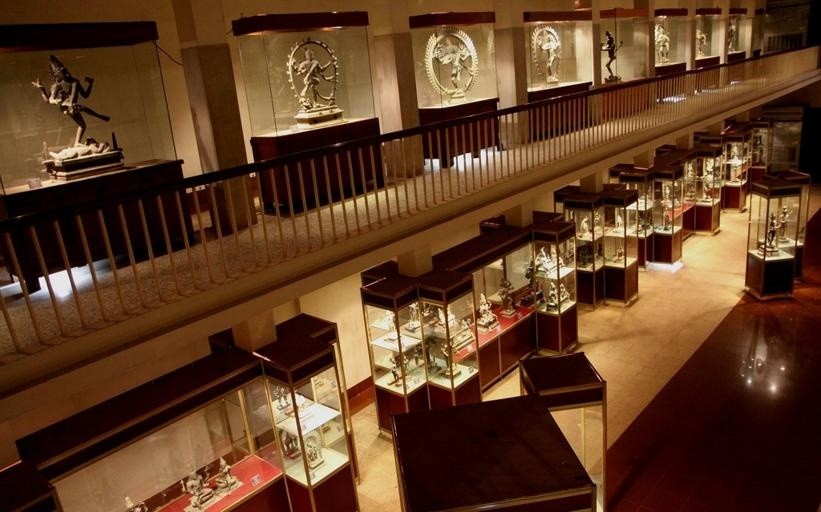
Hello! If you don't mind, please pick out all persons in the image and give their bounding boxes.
[32,54,110,147]
[297,48,332,107]
[477,277,571,319]
[539,21,738,83]
[768,204,794,248]
[124,457,239,512]
[436,42,473,89]
